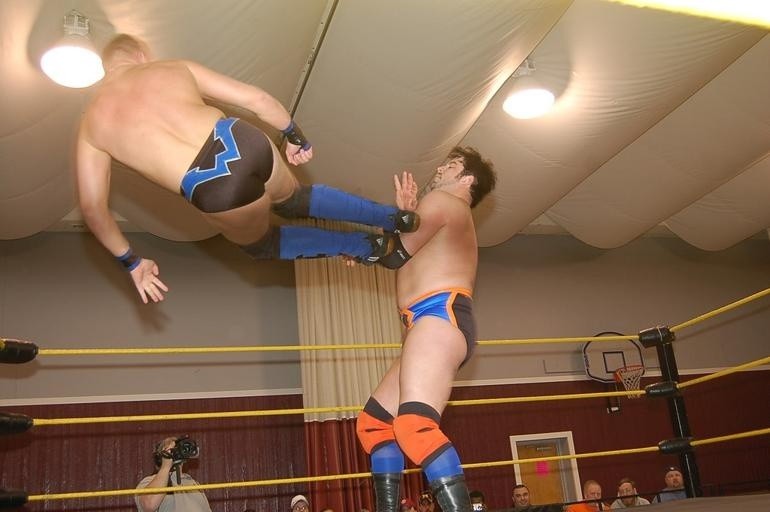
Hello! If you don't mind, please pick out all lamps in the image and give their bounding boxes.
[38,12,106,88]
[502,58,556,120]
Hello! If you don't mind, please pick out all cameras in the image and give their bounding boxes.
[472,503,483,511]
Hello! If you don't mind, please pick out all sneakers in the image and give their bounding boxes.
[362,235,395,266]
[391,210,419,233]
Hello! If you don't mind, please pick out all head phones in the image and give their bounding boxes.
[153,442,162,467]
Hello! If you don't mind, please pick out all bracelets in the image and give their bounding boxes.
[115,247,142,273]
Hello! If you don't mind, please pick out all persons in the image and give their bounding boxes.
[609,477,650,509]
[417,488,436,511]
[650,465,688,504]
[337,145,497,511]
[563,479,611,511]
[76,32,424,304]
[468,490,488,512]
[399,497,418,511]
[511,484,531,508]
[133,435,213,511]
[289,492,310,512]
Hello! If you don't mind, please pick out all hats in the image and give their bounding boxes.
[291,495,308,509]
[420,494,433,504]
[664,466,681,475]
